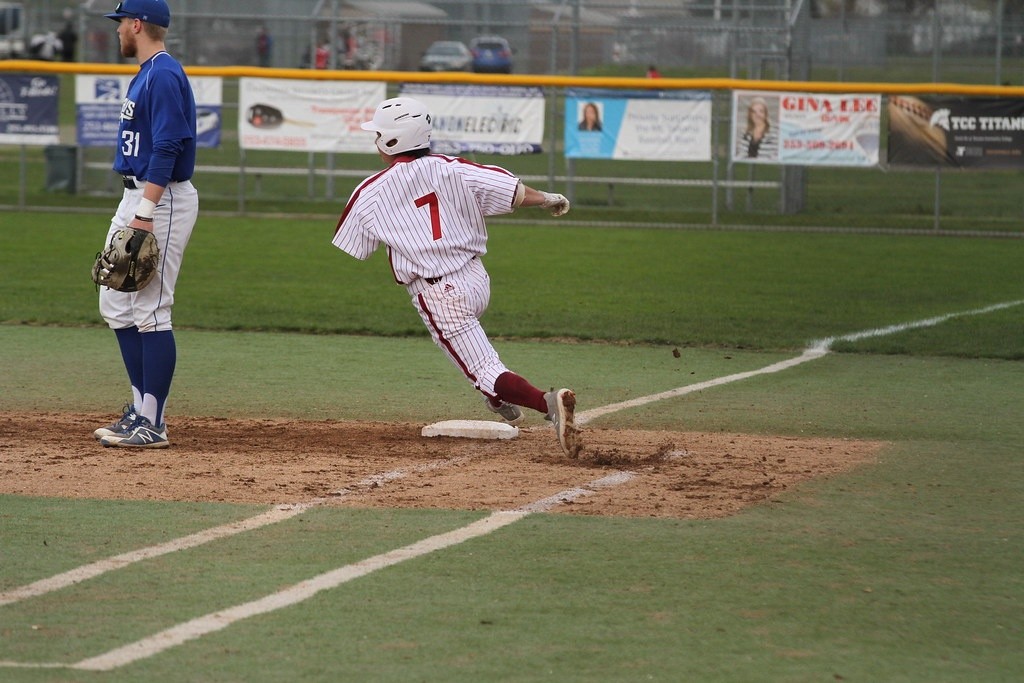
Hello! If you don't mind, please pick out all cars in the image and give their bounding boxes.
[420,41,473,71]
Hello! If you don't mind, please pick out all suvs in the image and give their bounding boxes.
[471,38,514,73]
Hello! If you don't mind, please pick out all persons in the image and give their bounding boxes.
[29,22,77,61]
[254,27,270,68]
[316,43,330,69]
[580,103,602,131]
[331,98,583,460]
[92,0,199,448]
[648,67,662,78]
[741,99,779,159]
[43,145,91,191]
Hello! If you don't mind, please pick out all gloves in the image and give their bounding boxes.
[538,191,570,217]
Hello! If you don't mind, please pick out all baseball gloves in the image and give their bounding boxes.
[91,225,160,294]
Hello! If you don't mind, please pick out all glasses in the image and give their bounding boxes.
[116,2,137,16]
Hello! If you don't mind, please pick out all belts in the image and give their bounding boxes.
[425,256,476,285]
[122,178,176,188]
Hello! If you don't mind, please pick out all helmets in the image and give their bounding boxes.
[361,97,432,156]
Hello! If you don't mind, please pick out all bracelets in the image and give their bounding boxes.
[135,214,152,222]
[137,197,156,217]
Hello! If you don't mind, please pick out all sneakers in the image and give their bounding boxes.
[484,398,525,425]
[100,415,169,448]
[543,386,581,459]
[93,400,138,440]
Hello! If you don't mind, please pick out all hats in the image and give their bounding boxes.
[103,0,170,27]
